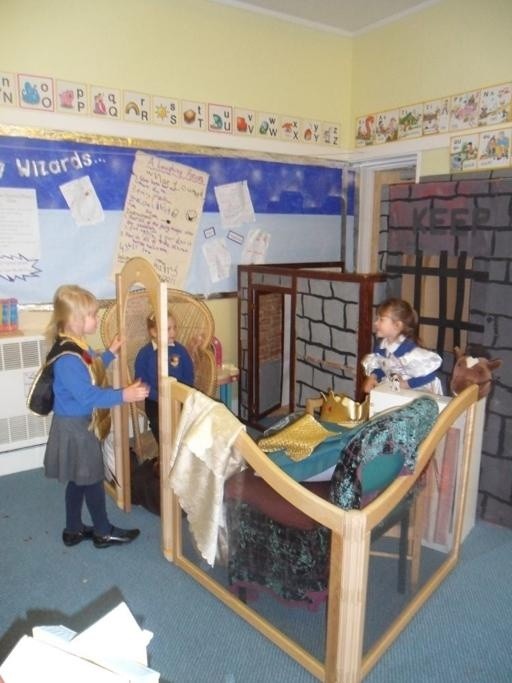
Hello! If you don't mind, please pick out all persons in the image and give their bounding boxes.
[133,311,192,444]
[362,295,442,394]
[42,283,151,547]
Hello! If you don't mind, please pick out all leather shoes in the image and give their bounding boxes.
[62,525,93,546]
[93,526,140,548]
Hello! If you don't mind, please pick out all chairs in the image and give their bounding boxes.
[100,288,217,463]
[221,392,439,605]
